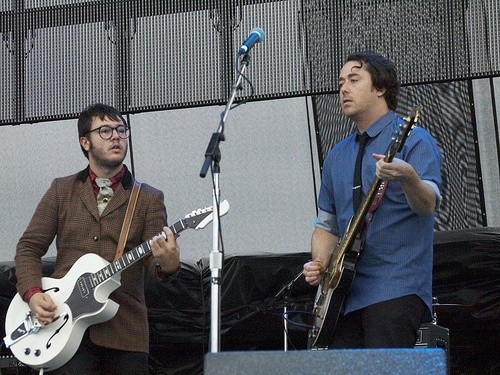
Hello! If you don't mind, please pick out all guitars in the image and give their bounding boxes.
[2,198,230,371]
[304,105,425,348]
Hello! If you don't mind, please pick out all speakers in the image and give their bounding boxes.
[205,347,447,375]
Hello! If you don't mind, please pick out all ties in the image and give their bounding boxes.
[353,131,369,216]
[95,177,114,216]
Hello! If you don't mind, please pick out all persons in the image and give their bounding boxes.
[14,103,180,375]
[303,50,443,348]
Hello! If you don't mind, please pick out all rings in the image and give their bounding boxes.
[34,313,40,319]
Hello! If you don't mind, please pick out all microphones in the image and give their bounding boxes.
[239,28,265,54]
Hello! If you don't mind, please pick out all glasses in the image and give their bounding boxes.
[83,125,131,140]
